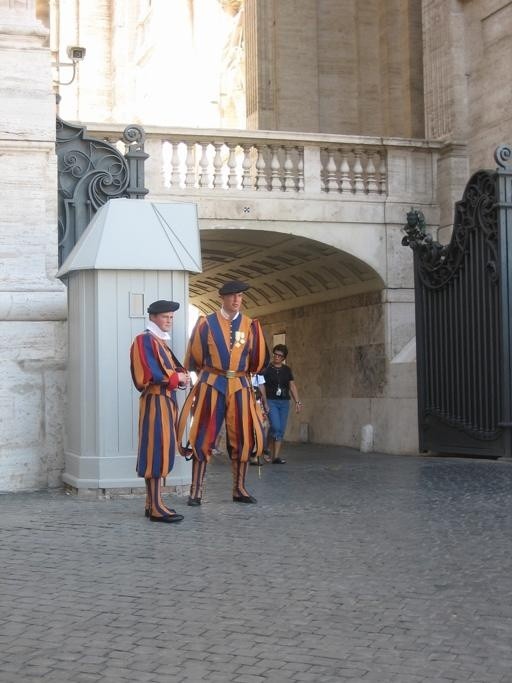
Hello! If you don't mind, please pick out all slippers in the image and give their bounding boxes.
[264,449,286,463]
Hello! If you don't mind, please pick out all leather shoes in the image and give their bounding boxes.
[233,495,257,503]
[145,508,184,521]
[187,496,201,505]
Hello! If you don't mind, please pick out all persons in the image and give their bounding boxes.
[249,373,273,463]
[261,344,303,465]
[129,301,191,522]
[176,280,267,507]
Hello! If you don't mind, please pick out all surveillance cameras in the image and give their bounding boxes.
[67,45,86,60]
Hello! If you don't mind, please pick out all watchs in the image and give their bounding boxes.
[296,401,302,404]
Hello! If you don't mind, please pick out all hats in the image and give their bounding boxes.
[219,281,249,294]
[148,300,179,312]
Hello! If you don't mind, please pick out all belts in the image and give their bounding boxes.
[205,365,245,378]
